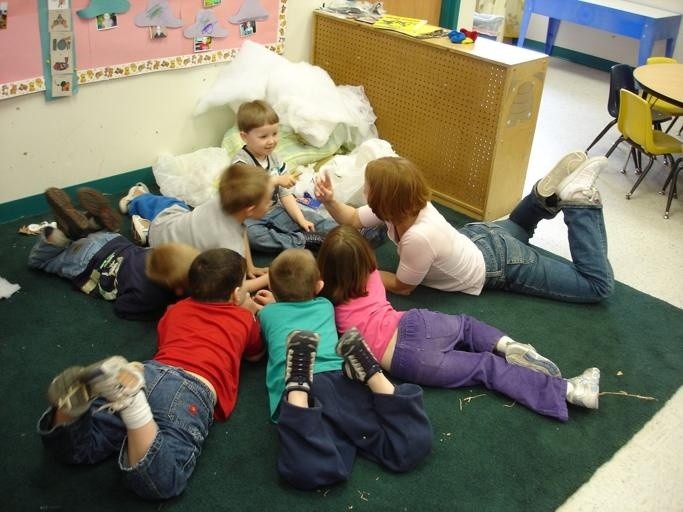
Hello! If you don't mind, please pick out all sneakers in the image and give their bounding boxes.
[566,366,601,410]
[360,225,388,251]
[75,186,123,233]
[302,230,327,251]
[504,342,562,380]
[74,353,150,414]
[335,325,381,386]
[282,329,321,388]
[42,185,91,239]
[119,180,155,246]
[552,155,611,203]
[534,150,589,199]
[45,365,102,416]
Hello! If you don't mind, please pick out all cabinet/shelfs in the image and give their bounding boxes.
[311,8,550,222]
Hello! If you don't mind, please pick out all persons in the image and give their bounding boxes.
[154,26,166,39]
[120,162,272,279]
[244,249,434,491]
[311,151,617,303]
[311,225,600,419]
[37,249,264,499]
[232,101,384,250]
[244,22,253,33]
[27,186,201,321]
[101,13,116,29]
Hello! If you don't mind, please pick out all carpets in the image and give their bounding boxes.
[0,190,682,512]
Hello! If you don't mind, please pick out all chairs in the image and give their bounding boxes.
[584,55,682,221]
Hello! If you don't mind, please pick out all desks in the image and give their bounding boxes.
[516,0,683,88]
